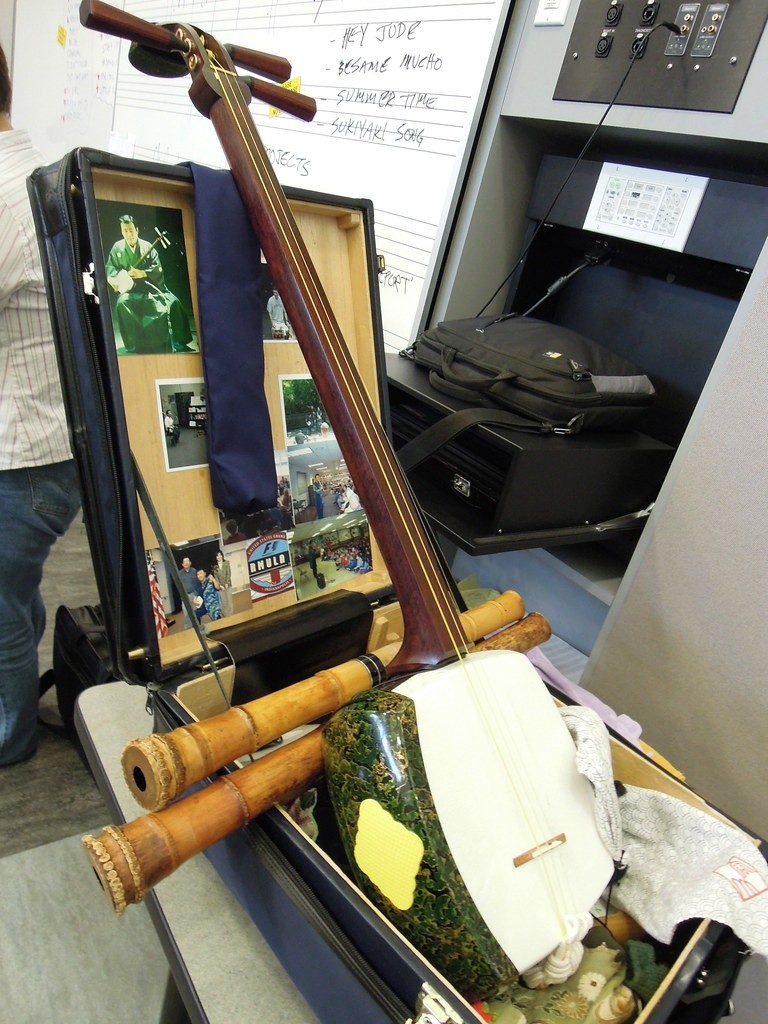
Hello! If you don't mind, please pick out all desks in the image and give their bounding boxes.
[72,682,768,1024]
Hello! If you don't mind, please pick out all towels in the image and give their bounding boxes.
[562,699,767,961]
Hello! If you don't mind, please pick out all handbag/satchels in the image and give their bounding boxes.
[395,313,662,473]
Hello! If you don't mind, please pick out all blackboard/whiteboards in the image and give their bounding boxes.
[9,1,516,354]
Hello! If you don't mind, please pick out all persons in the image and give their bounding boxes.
[178,555,200,629]
[223,476,296,545]
[211,549,234,619]
[192,567,221,625]
[312,472,364,515]
[294,412,336,444]
[0,40,85,769]
[164,409,181,447]
[267,286,293,340]
[308,535,373,589]
[105,214,196,355]
